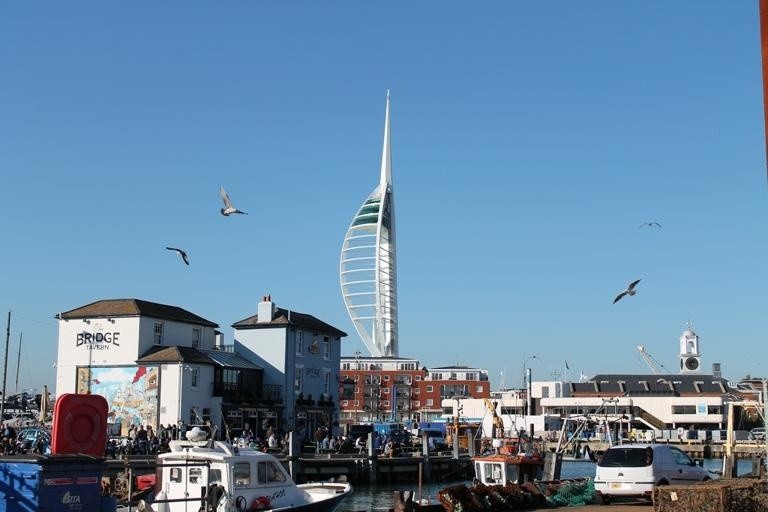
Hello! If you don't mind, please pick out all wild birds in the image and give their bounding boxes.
[613,279,642,304]
[219,184,248,216]
[165,246,190,266]
[639,221,663,229]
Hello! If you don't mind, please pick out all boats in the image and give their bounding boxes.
[472,435,543,484]
[117,421,354,512]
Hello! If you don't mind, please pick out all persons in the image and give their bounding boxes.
[105,420,397,458]
[1,422,18,455]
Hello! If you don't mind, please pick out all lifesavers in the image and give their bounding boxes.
[63,405,102,452]
[250,496,271,512]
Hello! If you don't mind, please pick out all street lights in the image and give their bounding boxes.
[523,355,543,387]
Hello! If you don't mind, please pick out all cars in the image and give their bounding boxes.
[416,428,444,445]
[748,427,767,440]
[15,428,50,451]
[593,443,714,505]
